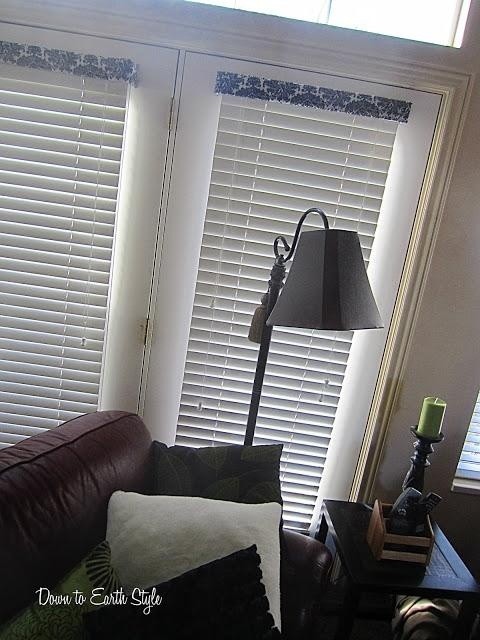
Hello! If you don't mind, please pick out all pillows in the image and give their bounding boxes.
[3,540,127,637]
[82,440,283,636]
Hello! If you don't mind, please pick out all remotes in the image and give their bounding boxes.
[402,491,442,529]
[389,487,423,529]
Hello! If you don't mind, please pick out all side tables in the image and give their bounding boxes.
[316,497,480,638]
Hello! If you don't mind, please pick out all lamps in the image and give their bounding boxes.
[237,203,384,446]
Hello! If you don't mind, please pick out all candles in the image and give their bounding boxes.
[415,394,447,439]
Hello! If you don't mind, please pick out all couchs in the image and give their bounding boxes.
[0,408,330,637]
